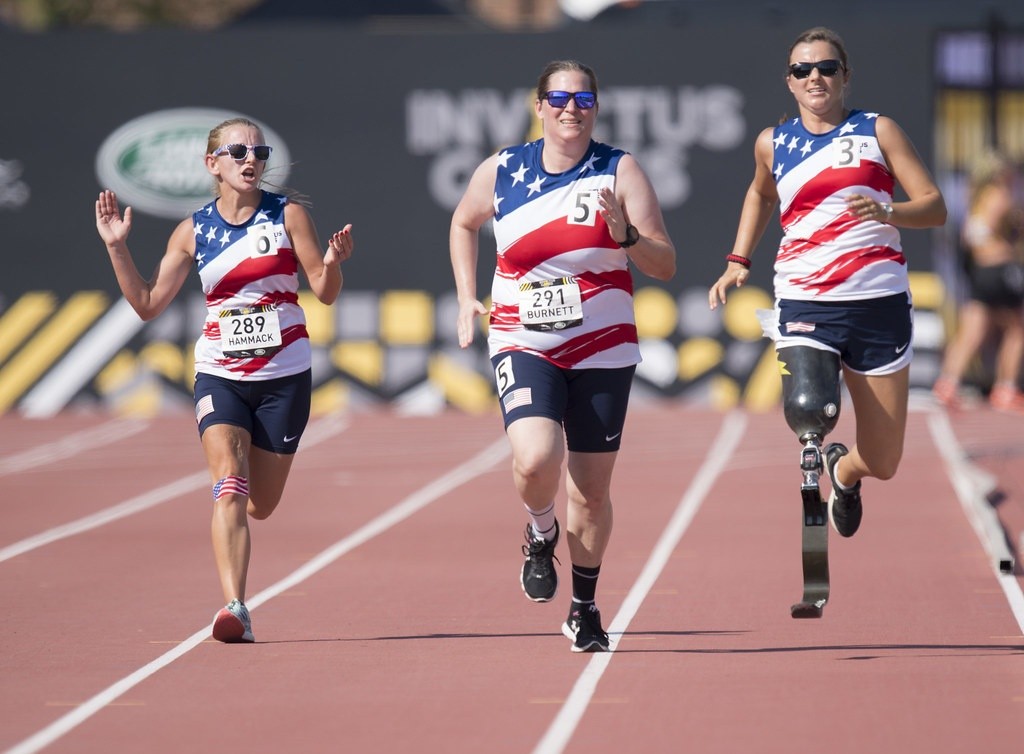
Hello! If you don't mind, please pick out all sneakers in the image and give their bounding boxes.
[822,443,863,538]
[212,598,255,642]
[562,606,609,653]
[520,515,561,602]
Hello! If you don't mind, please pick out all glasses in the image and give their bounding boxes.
[211,144,273,161]
[544,91,595,109]
[789,60,845,79]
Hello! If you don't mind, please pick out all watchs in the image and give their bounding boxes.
[617,222,639,248]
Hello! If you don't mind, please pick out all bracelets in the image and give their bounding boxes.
[879,202,894,226]
[725,253,751,268]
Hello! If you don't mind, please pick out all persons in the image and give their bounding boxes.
[710,28,947,618]
[935,159,1024,415]
[449,61,676,653]
[97,118,353,644]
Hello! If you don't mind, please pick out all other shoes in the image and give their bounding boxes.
[933,380,967,410]
[990,386,1024,415]
